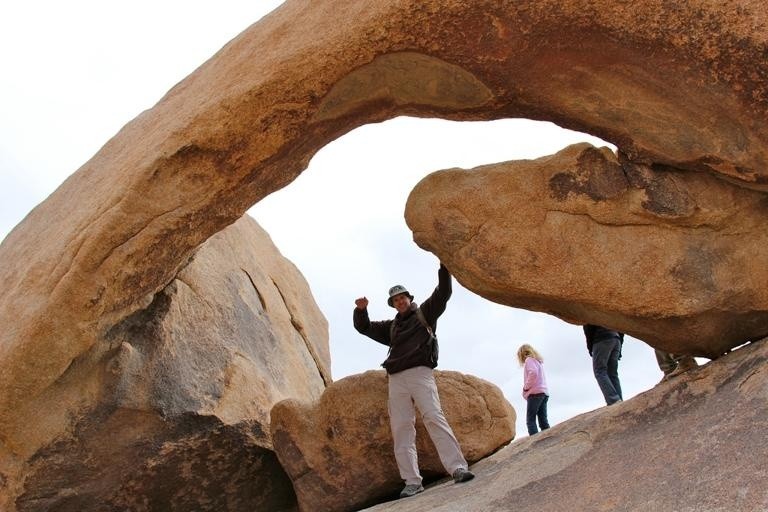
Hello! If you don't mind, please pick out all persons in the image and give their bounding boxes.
[583,324,625,403]
[654,346,699,385]
[352,258,477,496]
[517,343,550,435]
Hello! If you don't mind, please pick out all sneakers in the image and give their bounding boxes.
[453,468,475,483]
[400,484,425,497]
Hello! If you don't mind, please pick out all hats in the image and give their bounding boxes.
[388,285,414,307]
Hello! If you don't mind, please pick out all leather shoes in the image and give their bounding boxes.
[655,372,668,387]
[666,358,697,378]
